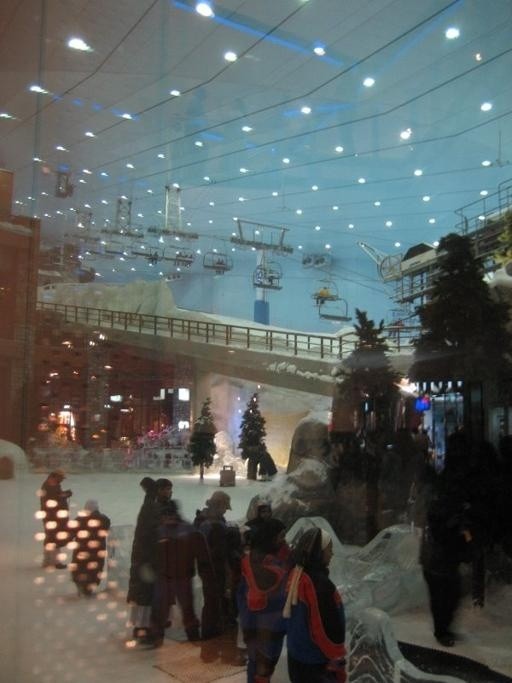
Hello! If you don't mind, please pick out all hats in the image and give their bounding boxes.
[52,467,68,480]
[211,490,232,510]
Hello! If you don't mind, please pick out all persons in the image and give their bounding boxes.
[388,320,403,338]
[46,497,111,599]
[316,287,327,305]
[40,470,74,570]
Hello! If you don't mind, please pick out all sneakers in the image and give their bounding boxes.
[132,617,200,650]
[432,624,457,648]
[186,628,247,666]
